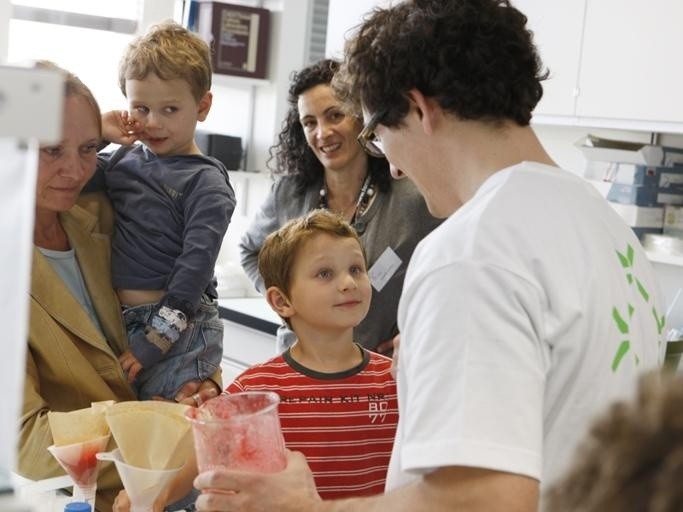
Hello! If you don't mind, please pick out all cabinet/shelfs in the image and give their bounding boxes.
[219,316,278,393]
[210,72,270,180]
[515,4,683,135]
[328,3,391,65]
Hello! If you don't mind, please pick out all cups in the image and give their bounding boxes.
[183,390,288,494]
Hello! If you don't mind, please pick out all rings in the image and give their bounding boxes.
[190,392,202,403]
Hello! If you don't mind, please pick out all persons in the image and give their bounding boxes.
[76,16,238,512]
[14,58,224,512]
[109,207,399,511]
[190,1,670,512]
[236,57,448,362]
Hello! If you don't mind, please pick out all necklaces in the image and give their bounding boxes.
[322,183,361,220]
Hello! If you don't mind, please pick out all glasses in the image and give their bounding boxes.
[356,102,398,159]
